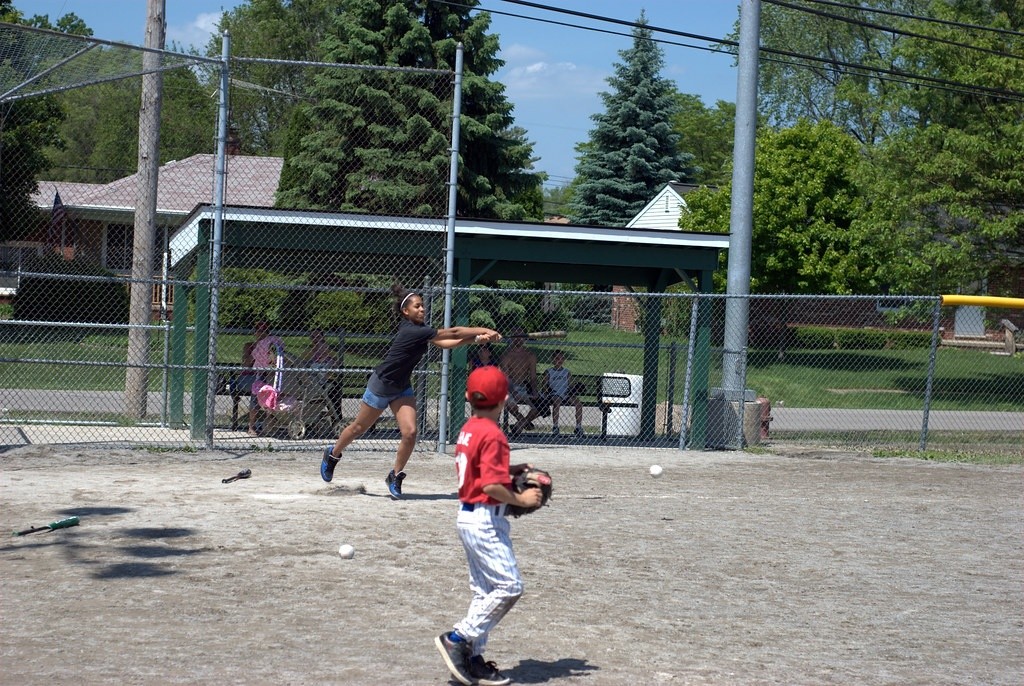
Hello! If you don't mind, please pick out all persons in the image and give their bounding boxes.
[462,343,498,399]
[433,365,543,686]
[298,328,338,380]
[501,322,540,436]
[542,350,584,437]
[320,285,504,499]
[237,323,270,434]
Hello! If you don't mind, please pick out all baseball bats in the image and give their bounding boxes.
[221,468,252,483]
[475,331,568,342]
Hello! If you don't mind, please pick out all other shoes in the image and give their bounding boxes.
[574,428,585,438]
[552,427,560,438]
[526,423,535,430]
[510,425,519,438]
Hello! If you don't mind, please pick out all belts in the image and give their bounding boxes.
[462,502,499,516]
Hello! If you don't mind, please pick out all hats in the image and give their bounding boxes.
[467,365,508,404]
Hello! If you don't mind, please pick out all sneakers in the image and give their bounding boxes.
[435,631,472,685]
[450,655,510,686]
[385,469,407,499]
[321,446,342,482]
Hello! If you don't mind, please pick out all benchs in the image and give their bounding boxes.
[500,373,637,439]
[211,362,343,432]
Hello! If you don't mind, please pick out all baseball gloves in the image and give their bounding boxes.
[514,468,552,517]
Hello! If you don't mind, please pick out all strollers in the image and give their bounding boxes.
[249,343,350,442]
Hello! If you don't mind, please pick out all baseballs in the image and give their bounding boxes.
[339,545,353,559]
[650,466,662,478]
